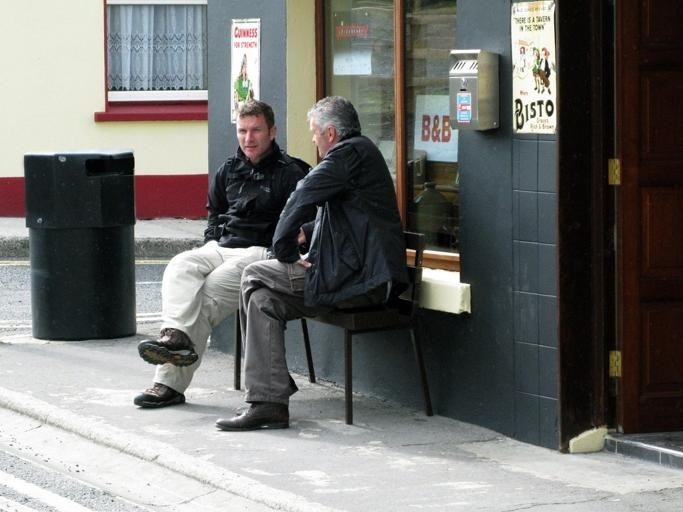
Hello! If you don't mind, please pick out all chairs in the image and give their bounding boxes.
[301,228,432,425]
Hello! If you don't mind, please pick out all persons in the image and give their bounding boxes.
[132,99,313,408]
[520,47,551,95]
[232,53,254,121]
[213,96,411,431]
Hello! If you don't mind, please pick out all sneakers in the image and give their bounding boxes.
[134,383,185,408]
[137,328,198,367]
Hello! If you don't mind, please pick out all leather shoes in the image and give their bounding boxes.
[235,375,299,415]
[215,402,289,431]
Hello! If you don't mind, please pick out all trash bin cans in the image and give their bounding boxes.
[22,150,139,342]
